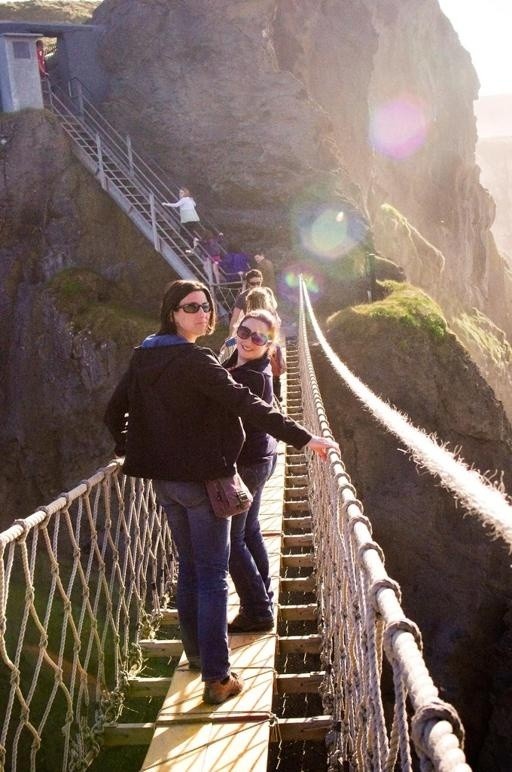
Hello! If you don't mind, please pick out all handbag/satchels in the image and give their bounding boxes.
[205,471,252,518]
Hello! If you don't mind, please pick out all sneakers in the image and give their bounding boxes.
[202,672,243,704]
[227,612,273,633]
[188,647,230,672]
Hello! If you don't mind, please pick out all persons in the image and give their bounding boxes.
[161,188,200,253]
[220,308,281,633]
[36,40,49,80]
[103,280,340,704]
[204,239,281,402]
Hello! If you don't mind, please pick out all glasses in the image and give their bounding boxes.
[248,282,262,285]
[174,302,210,313]
[237,326,268,345]
